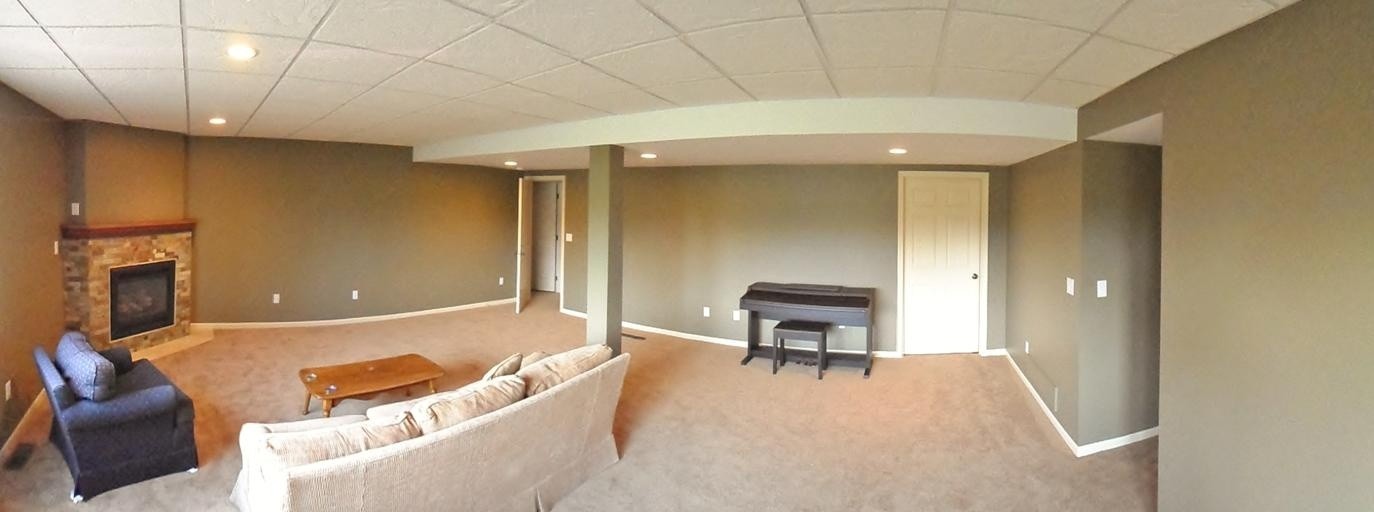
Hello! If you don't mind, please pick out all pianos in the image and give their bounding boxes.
[741,282,877,378]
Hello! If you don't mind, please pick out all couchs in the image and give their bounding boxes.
[238,340,634,508]
[32,329,199,505]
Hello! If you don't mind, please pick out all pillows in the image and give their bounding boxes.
[54,330,118,402]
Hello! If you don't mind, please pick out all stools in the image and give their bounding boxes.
[772,321,828,381]
